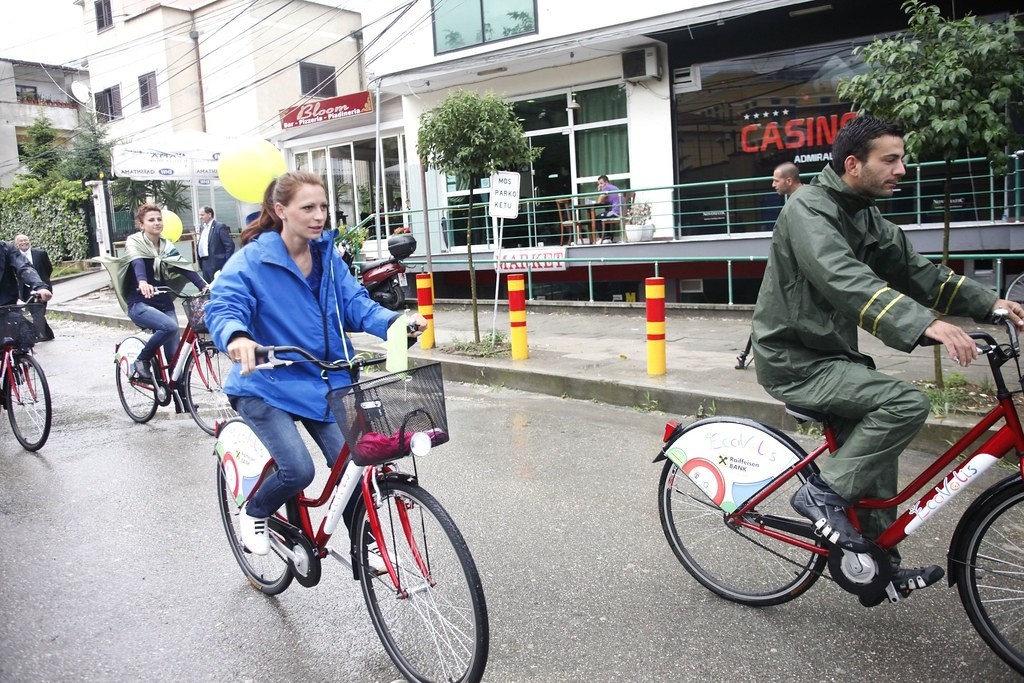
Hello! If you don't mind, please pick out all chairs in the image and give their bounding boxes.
[556,197,584,246]
[600,192,636,245]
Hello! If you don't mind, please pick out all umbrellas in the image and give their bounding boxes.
[110,128,225,220]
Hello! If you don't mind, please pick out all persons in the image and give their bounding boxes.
[770,162,801,196]
[196,206,235,285]
[750,116,1023,607]
[205,170,427,576]
[595,175,626,244]
[120,204,209,414]
[15,234,55,342]
[393,197,403,218]
[0,240,53,385]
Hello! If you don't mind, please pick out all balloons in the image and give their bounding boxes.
[217,134,288,205]
[159,210,184,244]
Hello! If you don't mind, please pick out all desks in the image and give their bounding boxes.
[326,214,348,229]
[568,202,610,244]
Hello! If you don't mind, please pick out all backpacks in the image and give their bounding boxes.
[0,308,37,345]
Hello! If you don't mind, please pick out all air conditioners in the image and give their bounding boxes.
[622,47,659,82]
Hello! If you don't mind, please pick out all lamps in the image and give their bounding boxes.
[569,52,581,109]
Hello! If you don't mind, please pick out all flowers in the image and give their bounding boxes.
[337,225,370,255]
[628,201,652,224]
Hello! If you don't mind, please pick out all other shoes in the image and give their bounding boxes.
[34,334,55,342]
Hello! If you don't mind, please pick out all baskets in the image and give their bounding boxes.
[0,302,48,346]
[325,362,450,467]
[182,294,211,333]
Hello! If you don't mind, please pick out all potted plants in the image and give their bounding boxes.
[359,186,372,228]
[321,179,344,227]
[17,93,38,104]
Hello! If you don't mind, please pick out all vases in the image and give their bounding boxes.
[625,224,655,242]
[45,100,78,108]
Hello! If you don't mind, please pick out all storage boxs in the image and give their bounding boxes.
[388,235,416,257]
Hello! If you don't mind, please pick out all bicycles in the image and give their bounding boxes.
[208,343,491,683]
[651,311,1024,676]
[0,298,53,453]
[114,284,235,436]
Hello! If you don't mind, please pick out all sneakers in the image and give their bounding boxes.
[239,501,271,555]
[174,402,198,413]
[596,238,613,245]
[133,360,153,379]
[789,473,870,553]
[368,541,390,572]
[859,565,944,608]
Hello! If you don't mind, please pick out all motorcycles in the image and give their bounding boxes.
[345,234,418,307]
